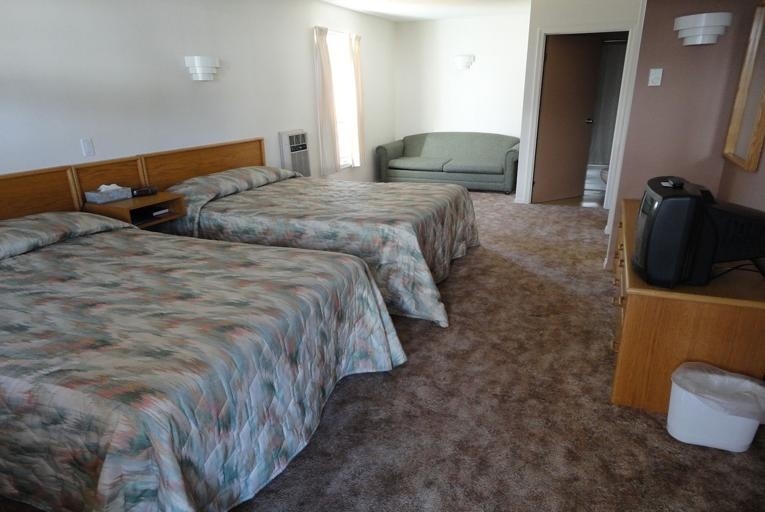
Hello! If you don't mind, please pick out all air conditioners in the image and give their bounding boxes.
[277,127,314,179]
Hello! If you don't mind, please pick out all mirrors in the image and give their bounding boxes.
[714,5,765,174]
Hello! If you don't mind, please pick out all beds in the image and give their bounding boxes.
[0,203,418,511]
[160,159,481,331]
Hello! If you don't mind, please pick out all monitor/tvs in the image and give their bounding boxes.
[631,176,765,289]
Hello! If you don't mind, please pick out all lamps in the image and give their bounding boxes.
[668,10,734,49]
[182,55,223,84]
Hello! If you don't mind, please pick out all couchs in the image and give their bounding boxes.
[372,131,520,195]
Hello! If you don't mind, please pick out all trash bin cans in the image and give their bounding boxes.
[667,361,765,453]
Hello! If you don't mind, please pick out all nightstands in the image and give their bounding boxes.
[79,183,190,236]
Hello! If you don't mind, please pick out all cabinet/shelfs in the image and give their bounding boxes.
[601,191,765,418]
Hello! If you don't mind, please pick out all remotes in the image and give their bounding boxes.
[669,177,684,186]
